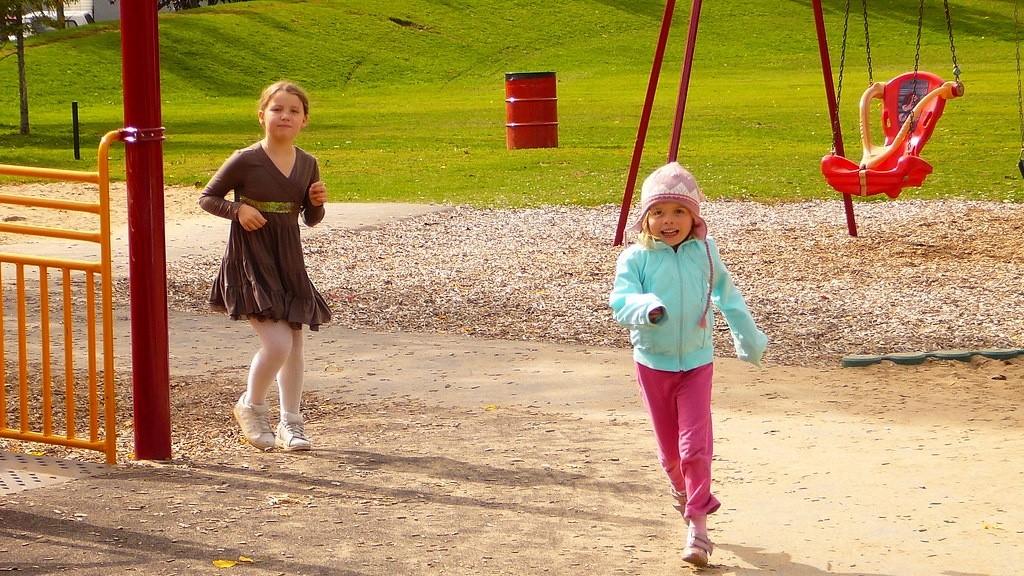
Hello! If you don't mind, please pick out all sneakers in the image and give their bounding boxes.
[276,422,310,450]
[682,529,713,566]
[671,486,688,516]
[234,391,275,451]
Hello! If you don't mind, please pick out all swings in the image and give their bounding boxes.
[1013,33,1024,179]
[819,1,965,202]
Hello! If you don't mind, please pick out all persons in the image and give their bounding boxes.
[199,81,332,449]
[608,162,767,564]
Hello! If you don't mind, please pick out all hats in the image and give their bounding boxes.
[627,162,708,245]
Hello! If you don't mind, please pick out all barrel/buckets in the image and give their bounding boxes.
[505,71,559,150]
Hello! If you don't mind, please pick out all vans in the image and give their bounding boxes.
[5,10,96,40]
